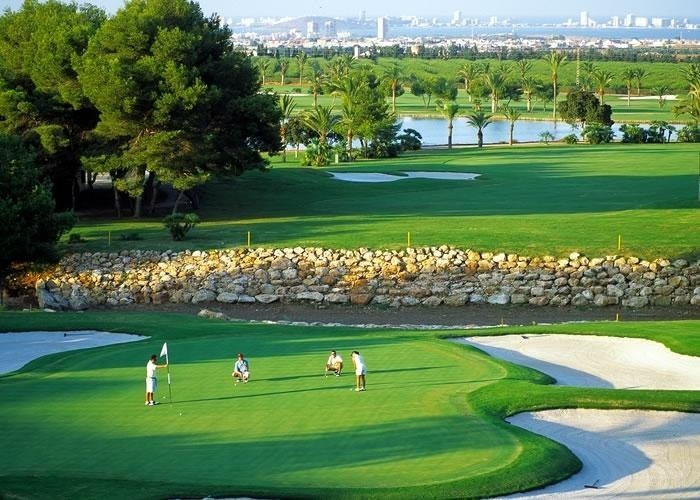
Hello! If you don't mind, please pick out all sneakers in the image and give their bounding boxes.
[243,379,248,383]
[336,373,341,376]
[236,378,241,382]
[360,387,366,391]
[355,388,360,392]
[334,370,338,375]
[150,401,159,405]
[145,402,154,405]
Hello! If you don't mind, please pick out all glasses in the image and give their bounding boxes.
[331,354,334,355]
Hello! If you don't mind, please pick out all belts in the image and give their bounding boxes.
[148,377,156,379]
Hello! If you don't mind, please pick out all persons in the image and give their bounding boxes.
[349,351,367,392]
[326,350,344,377]
[145,354,167,407]
[232,354,250,382]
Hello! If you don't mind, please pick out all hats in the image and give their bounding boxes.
[238,354,243,357]
[351,351,357,356]
